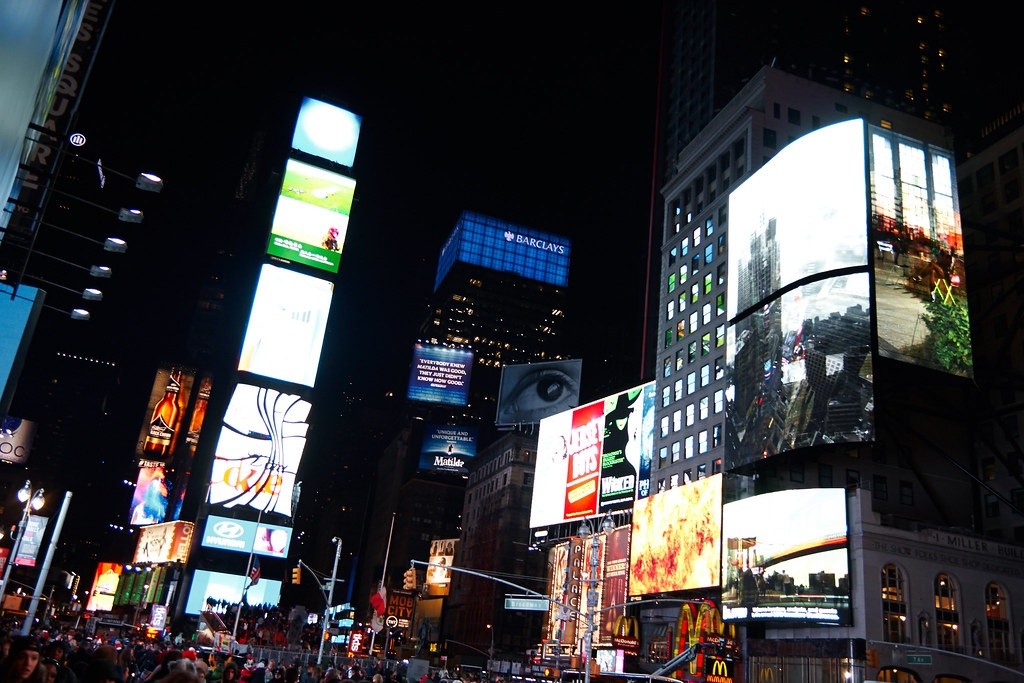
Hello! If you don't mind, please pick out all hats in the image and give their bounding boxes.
[350,666,359,671]
[9,636,41,654]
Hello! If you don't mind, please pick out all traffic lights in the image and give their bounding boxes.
[705,610,711,634]
[402,571,411,591]
[324,631,330,641]
[430,643,442,653]
[291,567,301,585]
[406,569,417,588]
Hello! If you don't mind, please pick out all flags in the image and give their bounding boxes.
[440,656,448,660]
[370,586,388,618]
[247,554,262,589]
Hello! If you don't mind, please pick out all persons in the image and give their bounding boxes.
[0,619,131,683]
[118,628,209,683]
[228,603,292,647]
[206,651,407,683]
[418,670,504,683]
[912,248,956,303]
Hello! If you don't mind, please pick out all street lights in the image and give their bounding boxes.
[577,509,615,683]
[314,536,343,665]
[487,624,493,683]
[0,480,49,599]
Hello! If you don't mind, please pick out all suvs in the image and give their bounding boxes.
[559,642,714,683]
